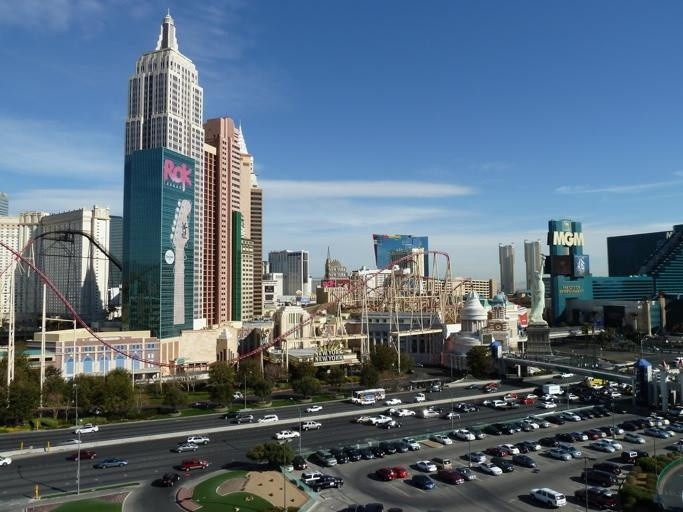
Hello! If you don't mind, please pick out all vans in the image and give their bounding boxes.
[530,487,568,508]
[180,458,209,471]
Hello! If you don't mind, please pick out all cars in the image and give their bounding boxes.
[232,414,254,425]
[55,437,81,445]
[0,455,12,467]
[161,473,180,488]
[233,392,243,400]
[306,405,323,413]
[411,371,680,491]
[174,442,198,453]
[301,421,322,431]
[575,486,617,509]
[193,402,209,409]
[274,430,300,440]
[352,387,415,430]
[293,434,420,493]
[92,456,128,470]
[257,414,278,424]
[74,425,98,434]
[222,411,240,420]
[72,448,96,461]
[185,435,210,445]
